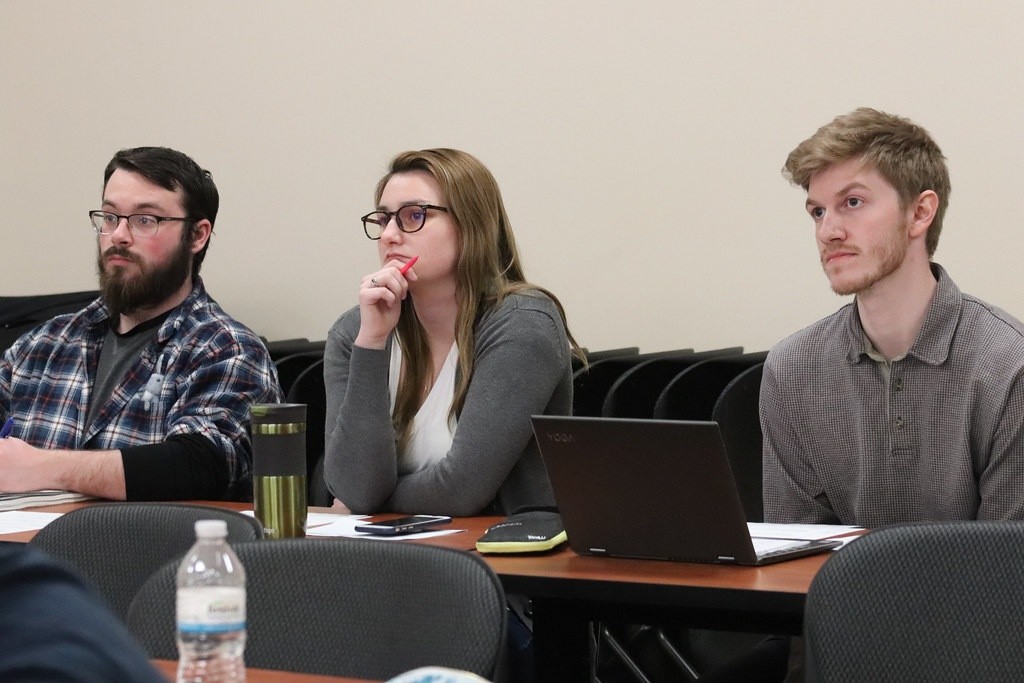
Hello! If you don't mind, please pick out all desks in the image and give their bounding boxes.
[2,489,873,683]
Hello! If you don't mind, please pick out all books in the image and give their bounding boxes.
[0,491,96,511]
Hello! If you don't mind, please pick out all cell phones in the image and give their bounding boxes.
[354,513,452,536]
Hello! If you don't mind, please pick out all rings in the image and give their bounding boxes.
[370,277,377,287]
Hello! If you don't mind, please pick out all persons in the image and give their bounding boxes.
[324,149,590,519]
[0,540,176,683]
[0,146,288,502]
[759,107,1023,526]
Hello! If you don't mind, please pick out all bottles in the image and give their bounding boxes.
[176,520,248,682]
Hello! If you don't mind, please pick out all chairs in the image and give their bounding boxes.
[126,536,511,682]
[805,521,1024,683]
[30,501,264,624]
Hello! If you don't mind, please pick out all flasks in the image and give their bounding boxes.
[251,403,307,540]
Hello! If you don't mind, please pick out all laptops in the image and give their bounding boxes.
[531,415,843,570]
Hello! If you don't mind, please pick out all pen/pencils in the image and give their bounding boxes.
[400,253,422,276]
